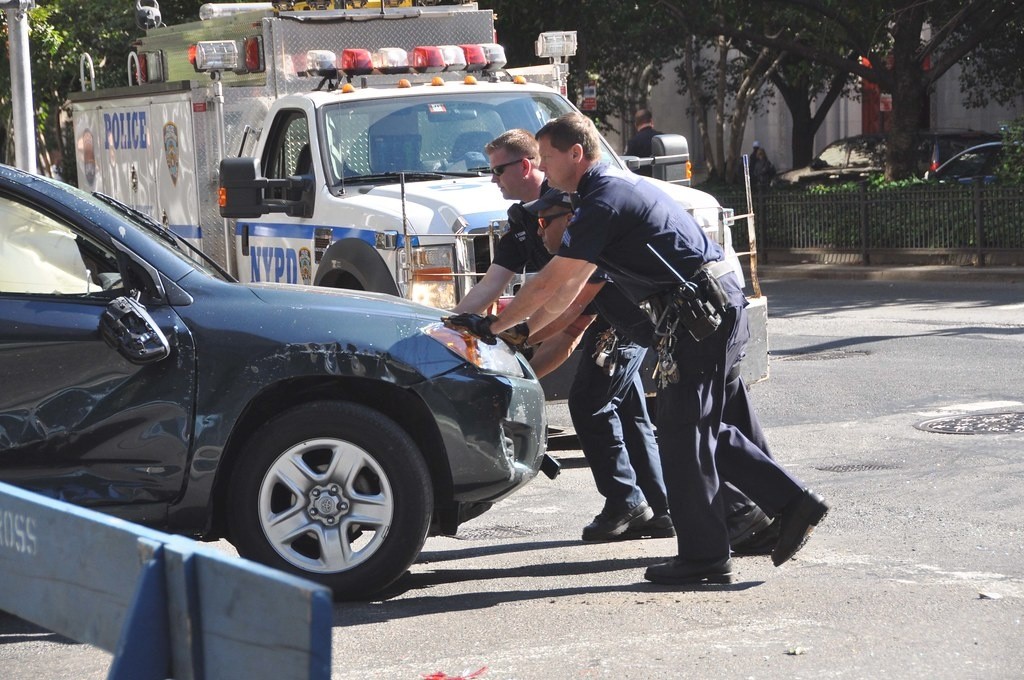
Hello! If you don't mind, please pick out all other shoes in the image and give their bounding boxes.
[731,517,782,554]
[727,504,771,545]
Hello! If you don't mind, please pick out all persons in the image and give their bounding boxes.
[749,140,775,187]
[447,128,677,538]
[624,108,666,177]
[480,187,783,553]
[444,112,829,584]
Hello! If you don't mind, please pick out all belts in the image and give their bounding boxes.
[698,258,735,280]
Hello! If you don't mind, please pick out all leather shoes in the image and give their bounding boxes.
[616,523,676,540]
[581,497,655,541]
[644,554,732,584]
[769,487,833,567]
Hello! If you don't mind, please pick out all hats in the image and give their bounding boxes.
[522,187,580,211]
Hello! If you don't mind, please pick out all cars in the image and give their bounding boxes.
[926,140,1024,189]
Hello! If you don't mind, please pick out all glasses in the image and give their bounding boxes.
[492,157,535,176]
[538,210,574,229]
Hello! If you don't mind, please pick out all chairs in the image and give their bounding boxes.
[295,143,362,178]
[451,130,495,162]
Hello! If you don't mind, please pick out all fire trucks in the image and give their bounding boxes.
[57,1,769,442]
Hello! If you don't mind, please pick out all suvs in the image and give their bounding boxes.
[772,125,1005,193]
[0,163,561,605]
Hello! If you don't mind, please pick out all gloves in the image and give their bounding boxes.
[440,312,497,346]
[496,320,529,347]
